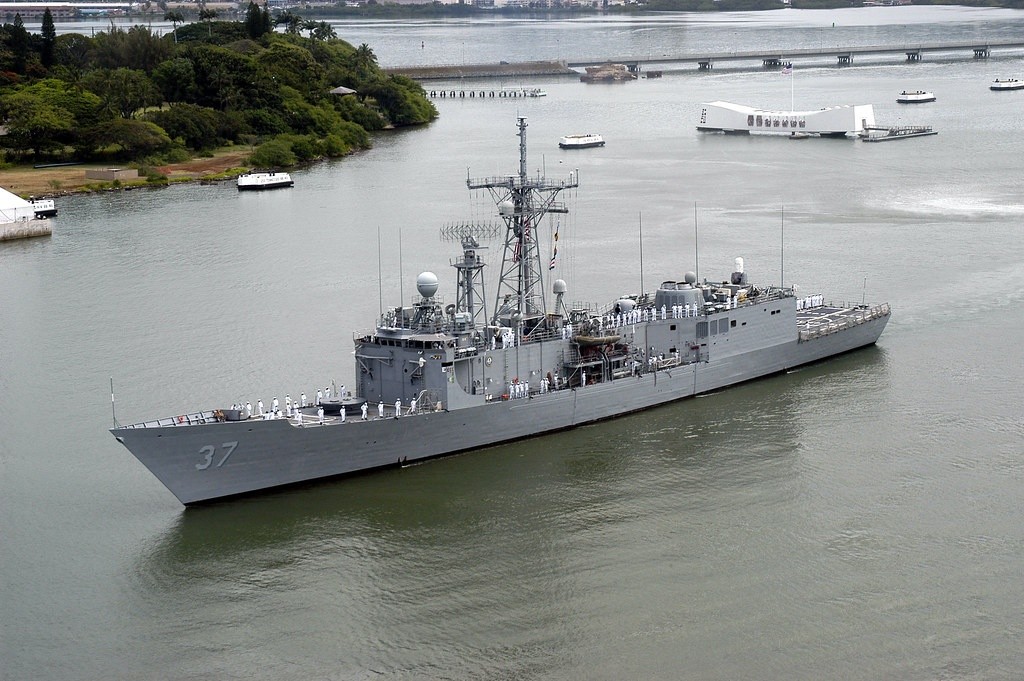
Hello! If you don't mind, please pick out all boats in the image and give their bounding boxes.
[238,170,294,191]
[573,334,621,347]
[989,79,1024,90]
[894,90,936,103]
[557,133,606,149]
[26,198,58,219]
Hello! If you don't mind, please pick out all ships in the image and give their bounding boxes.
[108,114,894,511]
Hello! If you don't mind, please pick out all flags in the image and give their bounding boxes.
[512,217,560,270]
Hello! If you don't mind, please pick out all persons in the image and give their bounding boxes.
[318,389,322,403]
[326,387,331,398]
[340,406,346,422]
[562,301,697,340]
[649,353,663,371]
[377,400,385,418]
[394,398,402,416]
[490,331,515,351]
[508,371,586,400]
[318,406,324,425]
[726,295,737,309]
[231,393,303,427]
[301,392,307,408]
[796,293,823,311]
[361,402,368,420]
[341,384,346,397]
[411,398,416,414]
